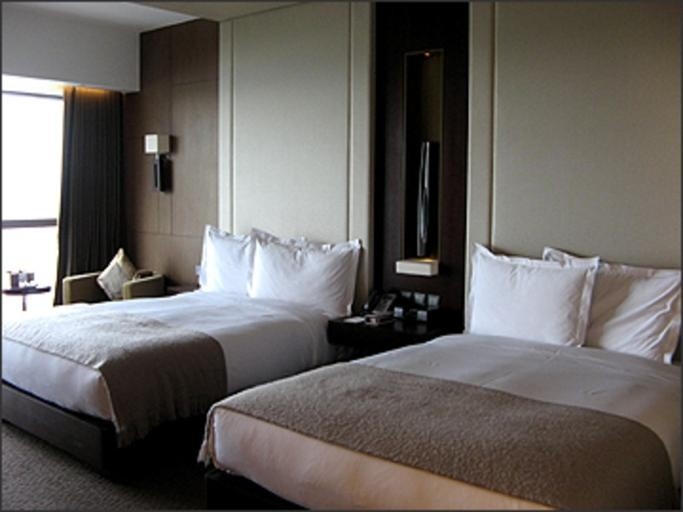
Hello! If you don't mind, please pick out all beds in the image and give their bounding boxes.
[2,291,343,477]
[198,333,681,510]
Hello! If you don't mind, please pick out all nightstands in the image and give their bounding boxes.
[327,311,460,361]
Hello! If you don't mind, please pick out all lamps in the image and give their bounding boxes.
[141,132,173,194]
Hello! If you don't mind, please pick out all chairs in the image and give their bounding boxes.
[60,270,166,304]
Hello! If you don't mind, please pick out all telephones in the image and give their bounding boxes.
[363,290,396,315]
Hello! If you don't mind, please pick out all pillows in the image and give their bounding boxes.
[194,223,362,318]
[97,247,137,301]
[464,240,682,364]
